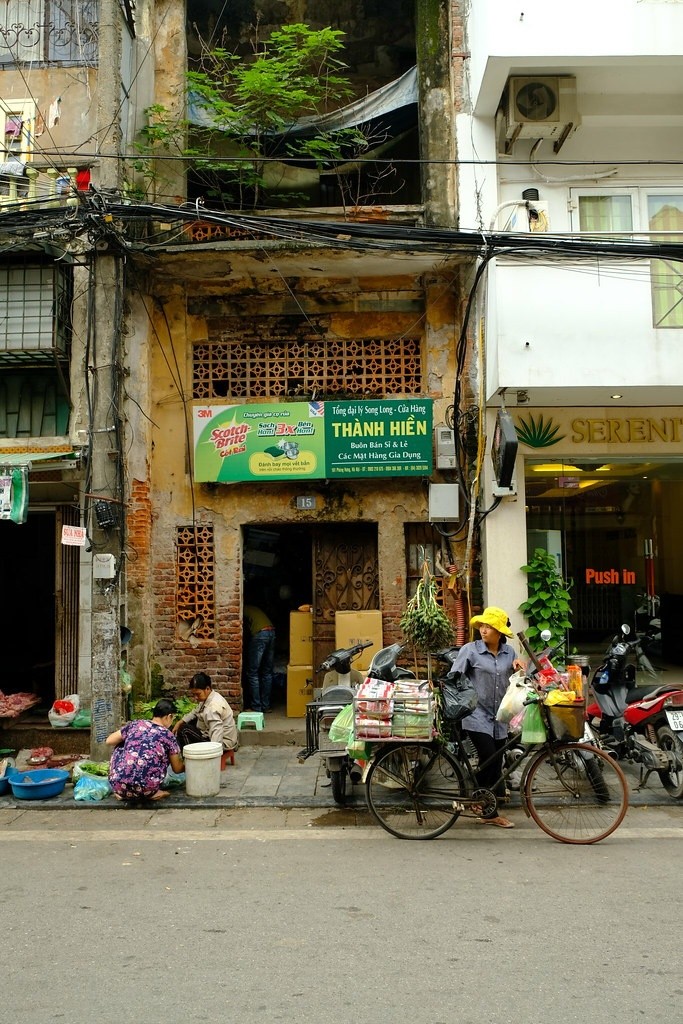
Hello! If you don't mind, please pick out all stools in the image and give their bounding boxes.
[237,712,267,732]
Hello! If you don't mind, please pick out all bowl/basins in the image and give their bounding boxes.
[8,768,70,799]
[0,767,19,795]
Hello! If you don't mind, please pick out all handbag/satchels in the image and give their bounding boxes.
[520,692,546,744]
[328,703,353,743]
[346,729,374,759]
[496,670,527,722]
[508,706,525,732]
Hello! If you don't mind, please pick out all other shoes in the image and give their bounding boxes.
[481,816,516,828]
[151,790,171,799]
[264,710,271,713]
[115,793,123,800]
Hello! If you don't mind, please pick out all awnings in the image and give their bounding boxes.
[0,452,73,525]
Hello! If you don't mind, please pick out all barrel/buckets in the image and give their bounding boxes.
[566,656,590,677]
[183,741,224,798]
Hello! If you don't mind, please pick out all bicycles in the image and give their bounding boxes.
[362,675,632,847]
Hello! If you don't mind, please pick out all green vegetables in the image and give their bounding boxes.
[130,694,198,727]
[73,761,110,784]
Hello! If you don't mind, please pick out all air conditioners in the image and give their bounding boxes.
[497,74,581,157]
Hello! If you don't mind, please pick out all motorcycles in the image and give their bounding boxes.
[522,629,610,804]
[585,621,683,800]
[295,637,375,804]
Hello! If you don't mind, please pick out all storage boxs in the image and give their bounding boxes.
[334,609,385,674]
[285,610,316,718]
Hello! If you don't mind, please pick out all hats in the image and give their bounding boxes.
[470,607,514,638]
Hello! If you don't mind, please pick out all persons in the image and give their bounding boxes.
[243,604,276,713]
[171,671,238,760]
[106,699,185,801]
[447,607,526,828]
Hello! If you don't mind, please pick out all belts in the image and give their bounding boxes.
[259,629,274,632]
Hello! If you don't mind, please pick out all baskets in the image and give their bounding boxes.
[549,703,586,743]
